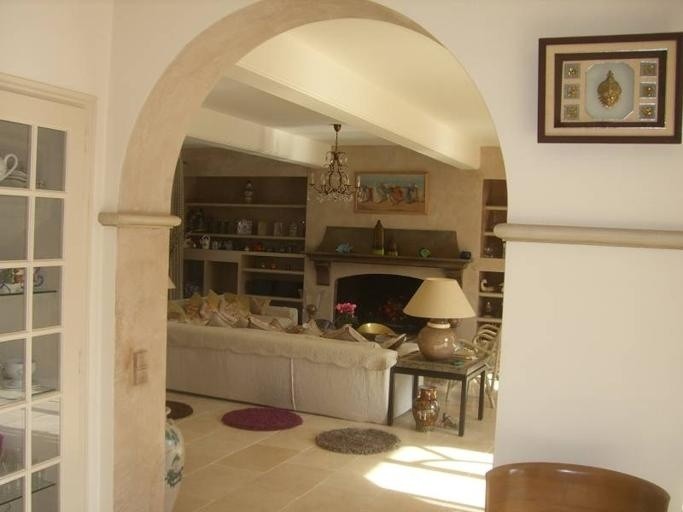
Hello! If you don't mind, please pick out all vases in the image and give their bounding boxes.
[166,406,185,511]
[334,314,360,330]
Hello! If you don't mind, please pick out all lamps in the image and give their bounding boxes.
[303,123,365,205]
[401,276,478,363]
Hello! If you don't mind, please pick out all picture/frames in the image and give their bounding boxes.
[353,170,429,217]
[536,30,683,144]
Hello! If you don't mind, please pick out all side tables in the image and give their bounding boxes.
[386,350,488,437]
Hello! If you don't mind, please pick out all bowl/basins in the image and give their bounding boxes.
[6,357,42,381]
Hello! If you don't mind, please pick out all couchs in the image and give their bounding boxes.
[167,320,426,426]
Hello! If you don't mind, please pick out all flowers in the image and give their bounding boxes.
[334,301,357,318]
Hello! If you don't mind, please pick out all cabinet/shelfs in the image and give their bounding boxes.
[299,224,464,288]
[1,87,89,511]
[477,271,505,342]
[481,178,505,261]
[184,174,307,300]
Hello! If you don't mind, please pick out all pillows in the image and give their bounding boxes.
[250,296,272,316]
[206,289,222,311]
[321,325,369,343]
[184,291,205,322]
[206,309,231,327]
[232,317,250,329]
[223,291,250,316]
[220,310,238,324]
[248,317,273,330]
[246,312,258,319]
[171,294,299,331]
[286,319,324,337]
[270,318,283,329]
[200,302,212,320]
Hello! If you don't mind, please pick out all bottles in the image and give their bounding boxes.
[242,180,253,204]
[165,406,185,512]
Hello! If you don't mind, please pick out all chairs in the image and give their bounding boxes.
[445,321,501,418]
[484,460,673,511]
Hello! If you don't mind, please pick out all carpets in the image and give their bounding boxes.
[221,407,303,431]
[166,399,195,420]
[316,427,401,454]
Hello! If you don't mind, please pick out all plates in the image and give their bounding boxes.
[2,281,46,291]
[1,377,37,387]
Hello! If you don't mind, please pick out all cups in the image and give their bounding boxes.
[0,148,18,185]
[198,235,210,249]
[5,450,53,487]
